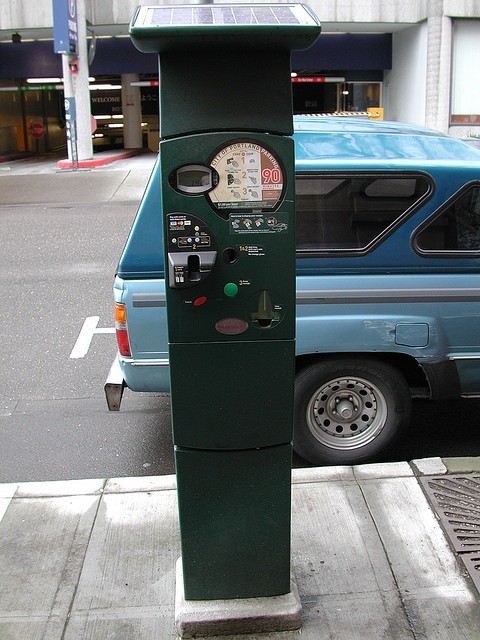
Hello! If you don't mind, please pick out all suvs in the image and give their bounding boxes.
[104,117,480,465]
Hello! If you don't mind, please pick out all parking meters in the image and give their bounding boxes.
[129,4,326,600]
